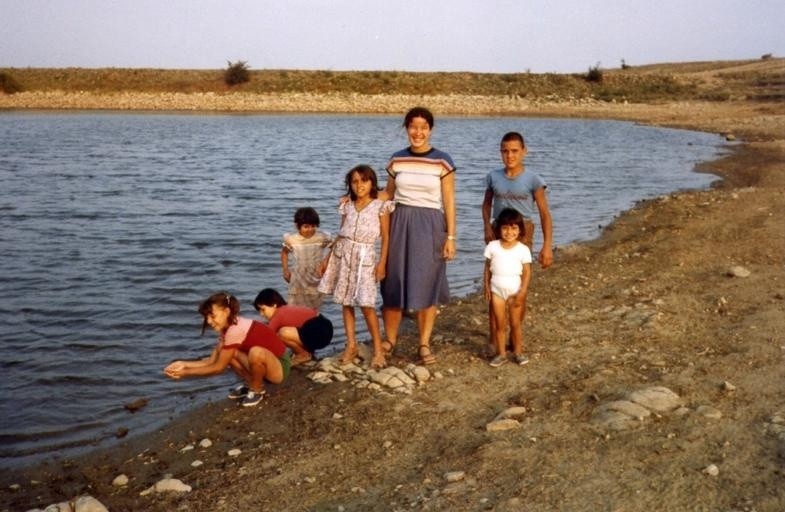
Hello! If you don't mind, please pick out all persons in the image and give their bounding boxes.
[339,107,457,365]
[280,206,334,312]
[316,163,390,368]
[482,132,554,357]
[481,208,534,368]
[162,292,292,408]
[253,288,334,367]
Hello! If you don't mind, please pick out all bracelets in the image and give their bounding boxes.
[445,234,456,241]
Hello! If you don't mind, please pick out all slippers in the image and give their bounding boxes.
[371,351,386,370]
[290,354,312,366]
[337,349,359,364]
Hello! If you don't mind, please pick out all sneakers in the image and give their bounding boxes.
[241,390,263,407]
[489,354,508,367]
[228,384,248,398]
[514,353,529,365]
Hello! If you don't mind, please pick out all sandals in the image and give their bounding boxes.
[418,345,436,365]
[381,339,394,359]
